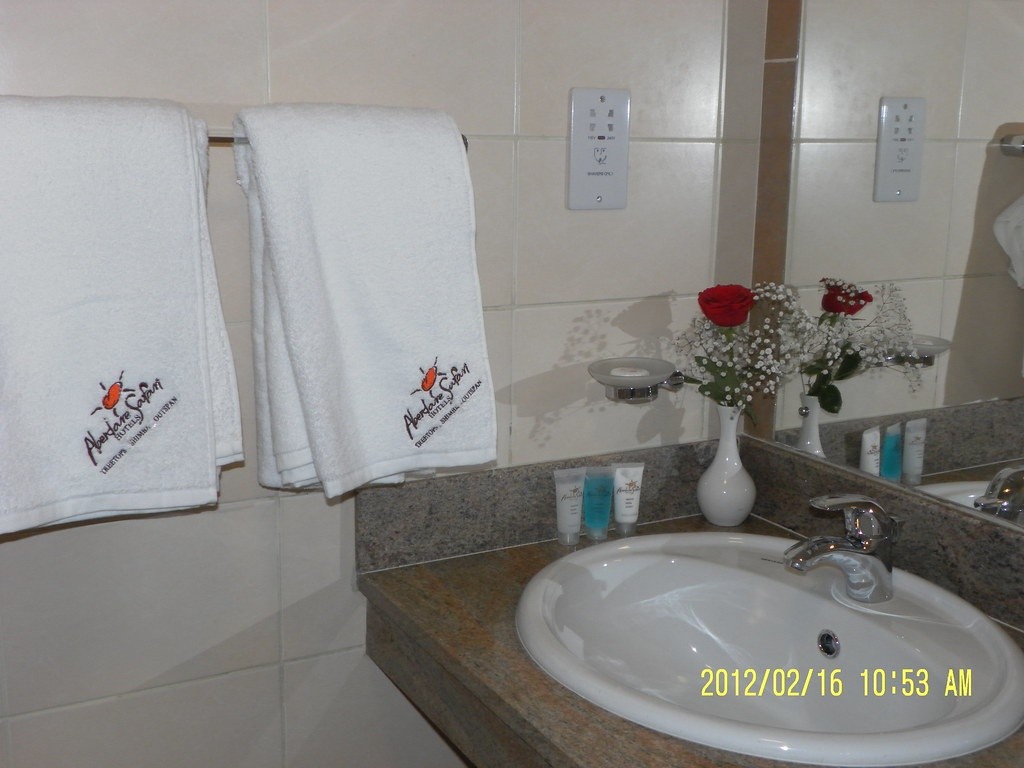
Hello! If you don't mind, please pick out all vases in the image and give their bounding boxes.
[696,405,757,527]
[797,393,826,458]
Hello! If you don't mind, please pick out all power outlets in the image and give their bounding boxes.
[874,96,925,203]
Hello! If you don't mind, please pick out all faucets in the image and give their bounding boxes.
[783,494,905,601]
[972,465,1024,523]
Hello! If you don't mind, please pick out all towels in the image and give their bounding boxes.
[0,93,245,534]
[236,101,496,500]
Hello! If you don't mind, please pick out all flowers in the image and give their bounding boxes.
[798,277,923,416]
[673,282,820,428]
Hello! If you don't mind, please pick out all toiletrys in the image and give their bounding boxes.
[860,417,927,485]
[551,464,644,544]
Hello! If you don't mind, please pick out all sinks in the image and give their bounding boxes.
[919,480,990,515]
[514,533,1023,768]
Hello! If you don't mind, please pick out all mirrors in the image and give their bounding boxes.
[742,0,1024,530]
